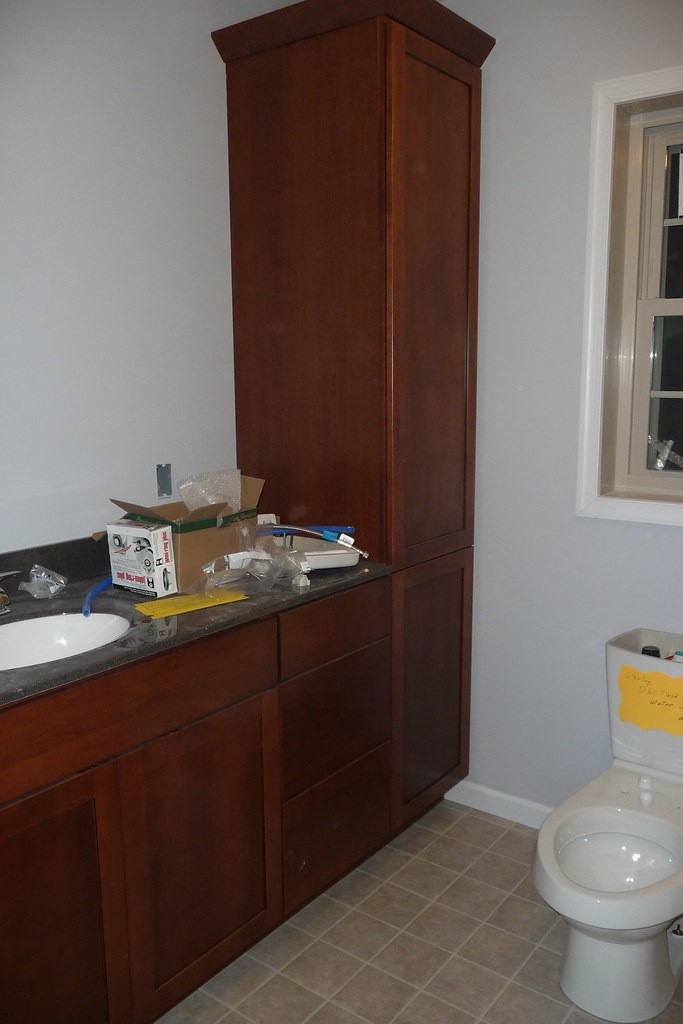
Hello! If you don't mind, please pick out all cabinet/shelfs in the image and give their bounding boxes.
[0,575,392,1024]
[209,0,495,840]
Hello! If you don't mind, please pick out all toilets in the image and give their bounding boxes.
[531,628,682,1023]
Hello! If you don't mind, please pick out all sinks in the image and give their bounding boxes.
[0,613,130,673]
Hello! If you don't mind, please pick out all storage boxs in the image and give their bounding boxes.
[106,521,178,599]
[109,474,266,595]
